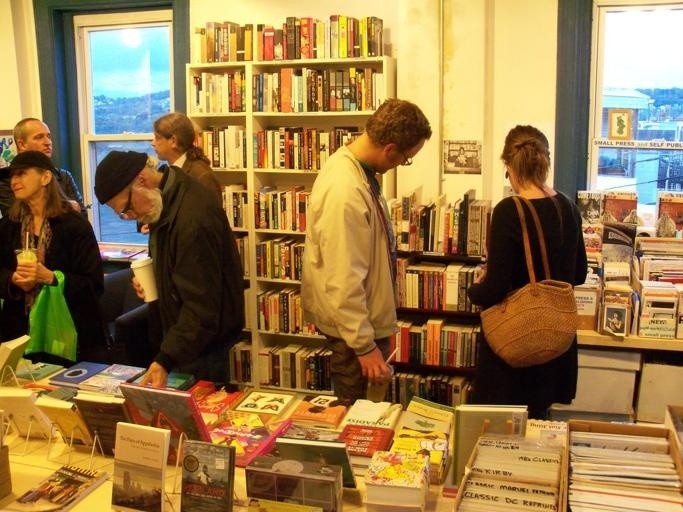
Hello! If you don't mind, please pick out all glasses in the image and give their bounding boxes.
[120,190,133,215]
[401,151,412,166]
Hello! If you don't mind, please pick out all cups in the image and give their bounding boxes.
[16,248,38,267]
[366,366,394,404]
[129,257,158,302]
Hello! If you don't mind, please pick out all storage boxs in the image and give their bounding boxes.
[454,346,683,511]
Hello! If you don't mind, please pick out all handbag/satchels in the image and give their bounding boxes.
[24,270,78,362]
[479,195,577,369]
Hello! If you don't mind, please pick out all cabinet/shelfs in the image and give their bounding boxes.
[186,55,492,410]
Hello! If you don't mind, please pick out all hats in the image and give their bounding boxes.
[94,151,148,205]
[4,150,54,173]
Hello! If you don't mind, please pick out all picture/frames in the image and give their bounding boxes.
[608,108,631,141]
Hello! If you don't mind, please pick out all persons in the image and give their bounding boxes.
[2,151,104,367]
[136,114,223,234]
[301,98,432,404]
[0,118,88,219]
[468,126,588,419]
[94,150,246,389]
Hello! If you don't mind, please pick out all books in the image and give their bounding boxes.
[573,190,683,338]
[1,335,683,512]
[387,185,512,368]
[254,67,377,336]
[191,72,252,329]
[201,14,383,63]
